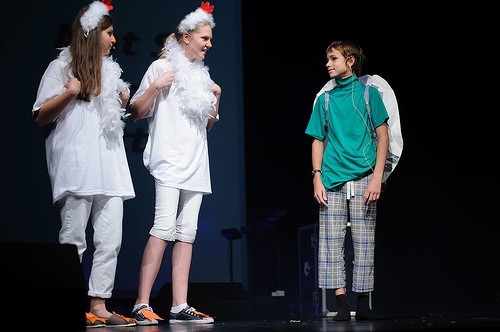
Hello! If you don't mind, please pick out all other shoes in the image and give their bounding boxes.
[332,304,351,321]
[355,304,384,321]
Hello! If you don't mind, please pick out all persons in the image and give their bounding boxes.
[30,0,136,328]
[130,8,221,324]
[305,39,390,321]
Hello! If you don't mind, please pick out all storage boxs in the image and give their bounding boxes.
[299,223,375,321]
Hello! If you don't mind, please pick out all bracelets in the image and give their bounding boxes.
[312,170,322,175]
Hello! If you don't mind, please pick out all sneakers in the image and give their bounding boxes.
[105,310,137,328]
[85,311,107,328]
[131,305,164,325]
[169,306,214,324]
